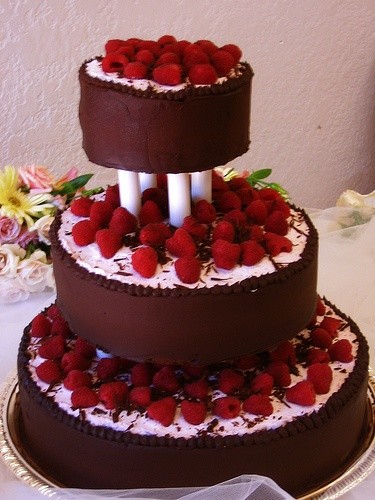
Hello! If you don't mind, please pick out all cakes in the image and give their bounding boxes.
[17,35,370,498]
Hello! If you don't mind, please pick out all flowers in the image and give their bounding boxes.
[1,163,96,305]
[337,190,371,227]
